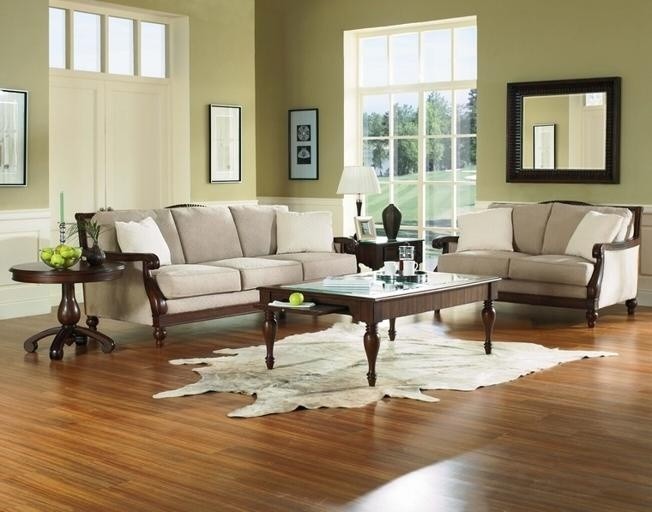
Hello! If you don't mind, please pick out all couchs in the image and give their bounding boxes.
[434,200,644,329]
[74,202,357,347]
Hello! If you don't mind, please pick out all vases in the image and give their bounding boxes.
[381,203,403,242]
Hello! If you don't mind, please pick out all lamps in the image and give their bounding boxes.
[336,166,383,240]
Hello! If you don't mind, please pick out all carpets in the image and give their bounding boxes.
[151,321,619,418]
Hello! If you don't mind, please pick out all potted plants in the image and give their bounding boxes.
[59,217,117,266]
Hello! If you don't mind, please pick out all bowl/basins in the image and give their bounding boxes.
[39,254,85,269]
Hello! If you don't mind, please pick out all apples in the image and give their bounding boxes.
[40,243,83,268]
[290,293,304,305]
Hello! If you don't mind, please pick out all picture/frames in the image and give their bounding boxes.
[208,103,241,183]
[0,88,30,189]
[288,108,319,181]
[533,122,557,169]
[354,216,377,241]
[583,92,605,108]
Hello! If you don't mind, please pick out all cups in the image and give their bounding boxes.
[384,262,398,275]
[399,246,415,274]
[402,260,419,276]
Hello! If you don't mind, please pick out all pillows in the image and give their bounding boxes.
[456,202,632,265]
[86,204,334,271]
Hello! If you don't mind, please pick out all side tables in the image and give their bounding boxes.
[8,259,126,361]
[340,237,425,273]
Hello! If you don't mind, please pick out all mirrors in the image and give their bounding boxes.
[505,77,622,185]
